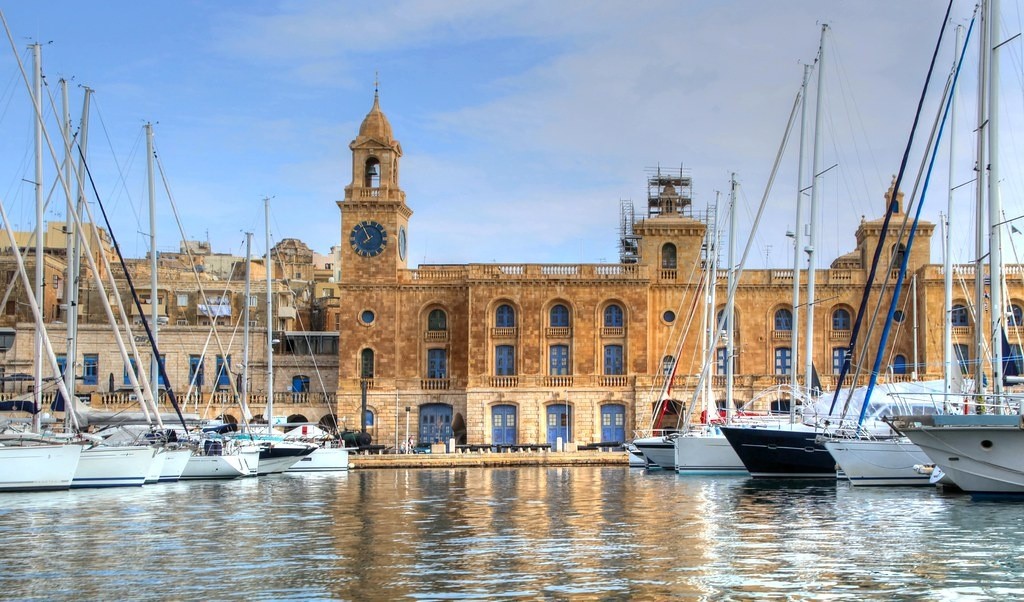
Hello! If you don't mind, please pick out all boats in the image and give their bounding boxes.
[616,0,1024,507]
[0,6,361,491]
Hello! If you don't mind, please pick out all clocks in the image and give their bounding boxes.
[349,220,388,257]
[398,225,406,261]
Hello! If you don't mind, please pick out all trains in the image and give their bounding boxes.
[335,418,386,455]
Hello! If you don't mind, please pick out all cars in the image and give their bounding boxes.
[414,442,433,455]
